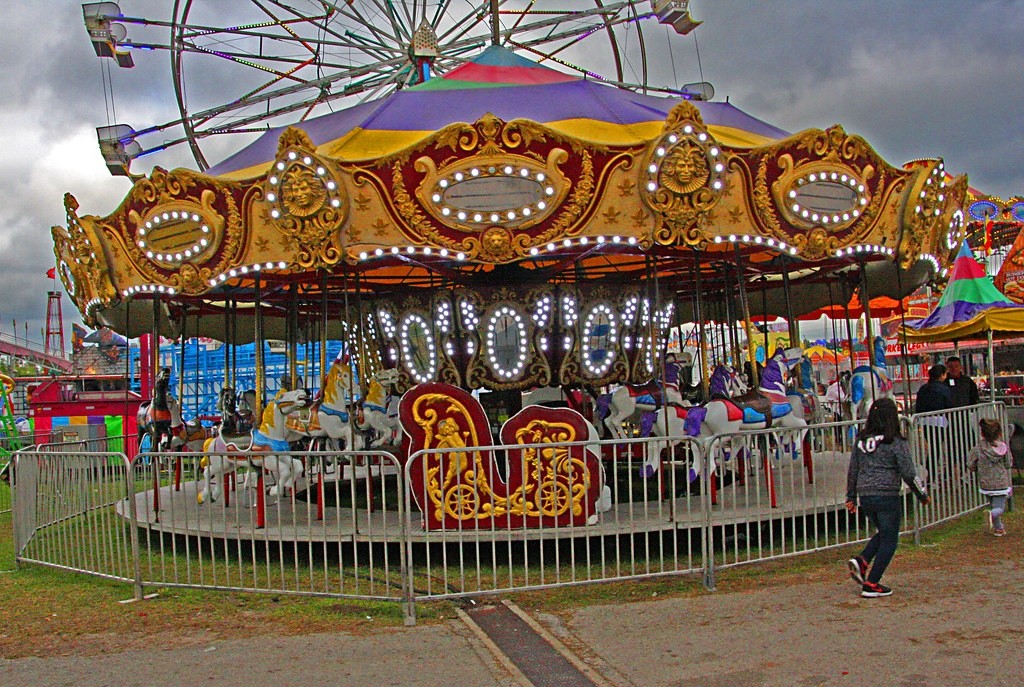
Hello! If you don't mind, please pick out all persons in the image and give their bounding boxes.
[915,357,981,491]
[966,417,1013,537]
[845,398,931,597]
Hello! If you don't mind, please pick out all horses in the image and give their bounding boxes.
[137,337,897,505]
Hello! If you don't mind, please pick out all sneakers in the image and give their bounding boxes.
[860,581,893,597]
[847,555,870,585]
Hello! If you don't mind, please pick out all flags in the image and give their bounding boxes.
[46,267,55,279]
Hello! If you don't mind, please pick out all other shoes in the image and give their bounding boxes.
[983,509,993,531]
[926,482,940,491]
[960,476,968,487]
[994,529,1007,537]
